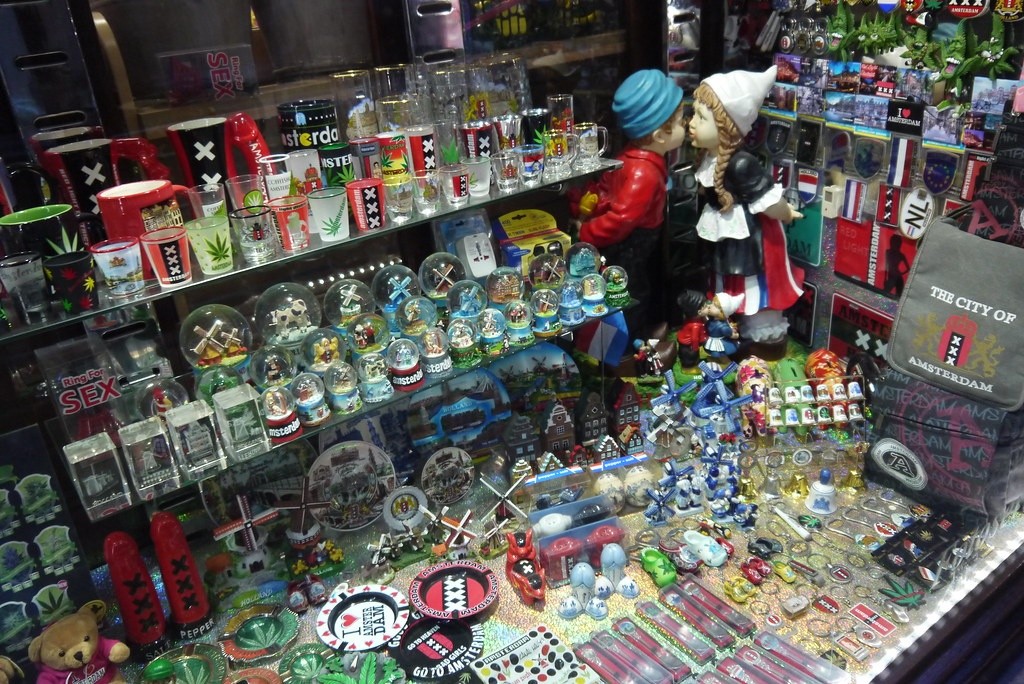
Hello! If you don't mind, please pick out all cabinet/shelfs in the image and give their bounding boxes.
[0,158,640,525]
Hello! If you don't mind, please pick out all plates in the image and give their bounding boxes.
[383,485,428,532]
[305,440,397,532]
[318,396,418,486]
[407,367,512,460]
[134,604,335,684]
[488,343,583,426]
[315,559,499,679]
[421,447,475,505]
[198,439,321,528]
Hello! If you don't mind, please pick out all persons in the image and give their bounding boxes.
[686,64,804,325]
[678,290,745,361]
[568,67,688,352]
[354,323,376,349]
[633,338,664,379]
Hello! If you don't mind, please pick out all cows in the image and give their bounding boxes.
[266,299,311,339]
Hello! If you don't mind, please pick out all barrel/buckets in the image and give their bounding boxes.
[90,0,260,108]
[250,0,374,77]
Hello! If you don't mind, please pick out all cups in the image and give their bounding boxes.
[346,118,496,235]
[166,112,274,220]
[542,93,610,179]
[330,52,534,143]
[275,98,339,173]
[224,147,324,265]
[96,179,202,283]
[0,154,56,216]
[140,226,192,288]
[0,203,97,313]
[184,171,234,278]
[491,109,555,193]
[0,293,23,334]
[29,125,173,251]
[89,236,146,295]
[307,141,359,243]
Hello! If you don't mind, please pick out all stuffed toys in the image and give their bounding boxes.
[28,600,129,684]
